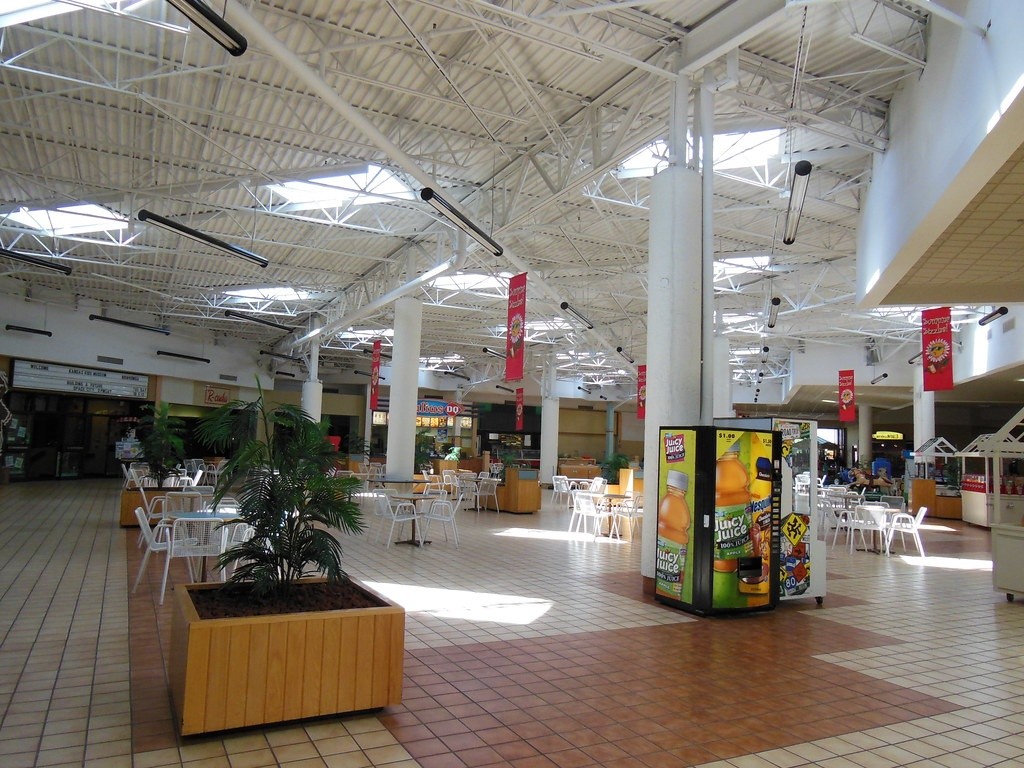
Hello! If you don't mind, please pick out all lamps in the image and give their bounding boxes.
[89,314,171,336]
[871,373,888,384]
[0,236,72,276]
[276,371,295,378]
[224,310,293,335]
[138,209,270,270]
[260,350,294,361]
[363,349,392,360]
[5,304,53,337]
[165,0,248,58]
[908,351,922,364]
[354,370,386,380]
[157,350,210,364]
[420,160,813,403]
[979,306,1008,326]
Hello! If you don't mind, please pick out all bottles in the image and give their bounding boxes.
[656,470,690,600]
[714,438,754,573]
[964,472,985,483]
[738,457,772,593]
[1000,475,1024,495]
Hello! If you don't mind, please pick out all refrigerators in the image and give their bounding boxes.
[657,425,783,619]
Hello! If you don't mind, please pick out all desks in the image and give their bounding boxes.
[172,494,218,538]
[460,478,502,512]
[390,493,440,547]
[145,473,184,487]
[577,492,631,538]
[196,461,218,485]
[857,508,907,558]
[828,494,865,532]
[168,511,242,582]
[564,478,594,508]
[452,473,472,501]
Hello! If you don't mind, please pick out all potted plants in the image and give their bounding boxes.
[479,450,531,512]
[118,400,186,528]
[169,373,406,746]
[598,453,632,507]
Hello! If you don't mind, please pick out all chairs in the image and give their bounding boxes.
[128,458,928,607]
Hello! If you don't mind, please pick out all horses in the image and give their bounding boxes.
[846,468,894,494]
